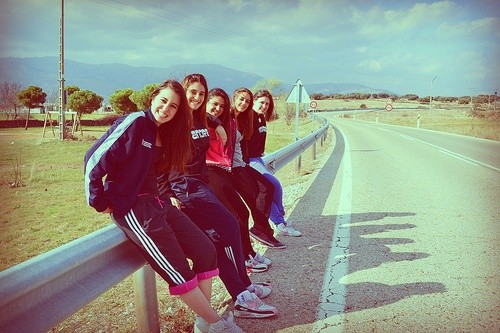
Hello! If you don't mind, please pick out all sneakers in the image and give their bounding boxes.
[233,289,277,319]
[193,309,245,333]
[245,252,273,273]
[249,223,287,249]
[275,222,302,236]
[249,282,273,299]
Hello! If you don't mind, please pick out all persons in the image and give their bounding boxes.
[246,88,303,237]
[229,88,287,249]
[84,80,246,333]
[156,75,278,319]
[206,89,272,272]
